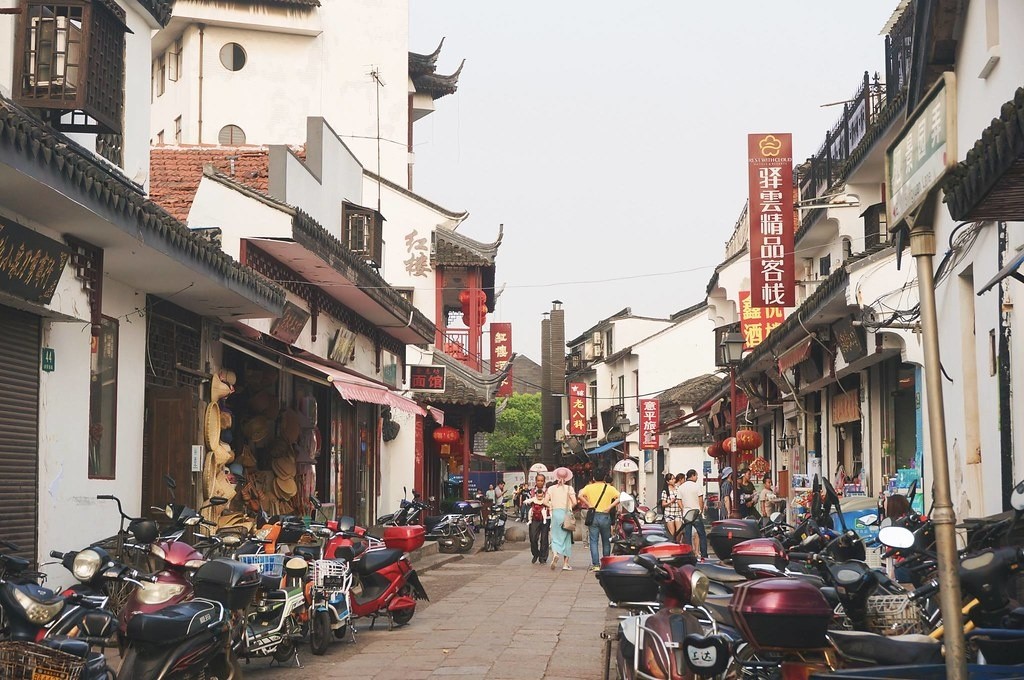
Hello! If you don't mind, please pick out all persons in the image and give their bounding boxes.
[577,469,621,572]
[661,469,708,558]
[476,480,531,523]
[523,467,577,570]
[760,478,776,527]
[719,467,761,520]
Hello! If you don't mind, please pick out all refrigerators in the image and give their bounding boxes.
[827,496,879,544]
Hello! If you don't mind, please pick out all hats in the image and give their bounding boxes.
[731,471,745,481]
[211,373,230,403]
[553,466,573,488]
[721,466,733,479]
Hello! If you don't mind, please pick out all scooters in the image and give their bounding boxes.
[595,479,1024,680]
[377,486,476,554]
[0,495,430,680]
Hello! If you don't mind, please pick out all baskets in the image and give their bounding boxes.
[200,401,301,537]
[237,554,285,589]
[833,595,924,637]
[0,641,87,680]
[308,559,353,595]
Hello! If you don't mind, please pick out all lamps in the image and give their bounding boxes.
[788,428,800,448]
[777,433,787,451]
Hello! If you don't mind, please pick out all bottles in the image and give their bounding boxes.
[793,491,825,513]
[903,465,907,469]
[844,484,861,492]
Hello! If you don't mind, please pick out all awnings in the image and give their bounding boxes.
[226,332,444,426]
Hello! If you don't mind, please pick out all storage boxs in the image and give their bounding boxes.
[191,559,261,610]
[500,511,508,522]
[467,502,484,514]
[383,525,425,552]
[619,514,635,524]
[595,555,658,602]
[268,516,305,541]
[452,501,471,515]
[730,577,835,649]
[639,541,697,567]
[707,519,762,562]
[731,536,789,576]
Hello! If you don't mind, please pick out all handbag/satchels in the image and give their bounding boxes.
[562,513,576,531]
[585,508,595,526]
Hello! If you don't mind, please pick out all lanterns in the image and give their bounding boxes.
[722,437,742,455]
[708,445,722,463]
[432,425,460,454]
[737,429,761,460]
[458,287,488,327]
[437,435,471,474]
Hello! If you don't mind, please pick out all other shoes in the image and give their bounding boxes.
[540,559,546,564]
[563,563,573,571]
[532,550,540,563]
[550,555,559,570]
[589,564,600,571]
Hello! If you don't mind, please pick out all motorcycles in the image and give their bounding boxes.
[484,506,507,551]
